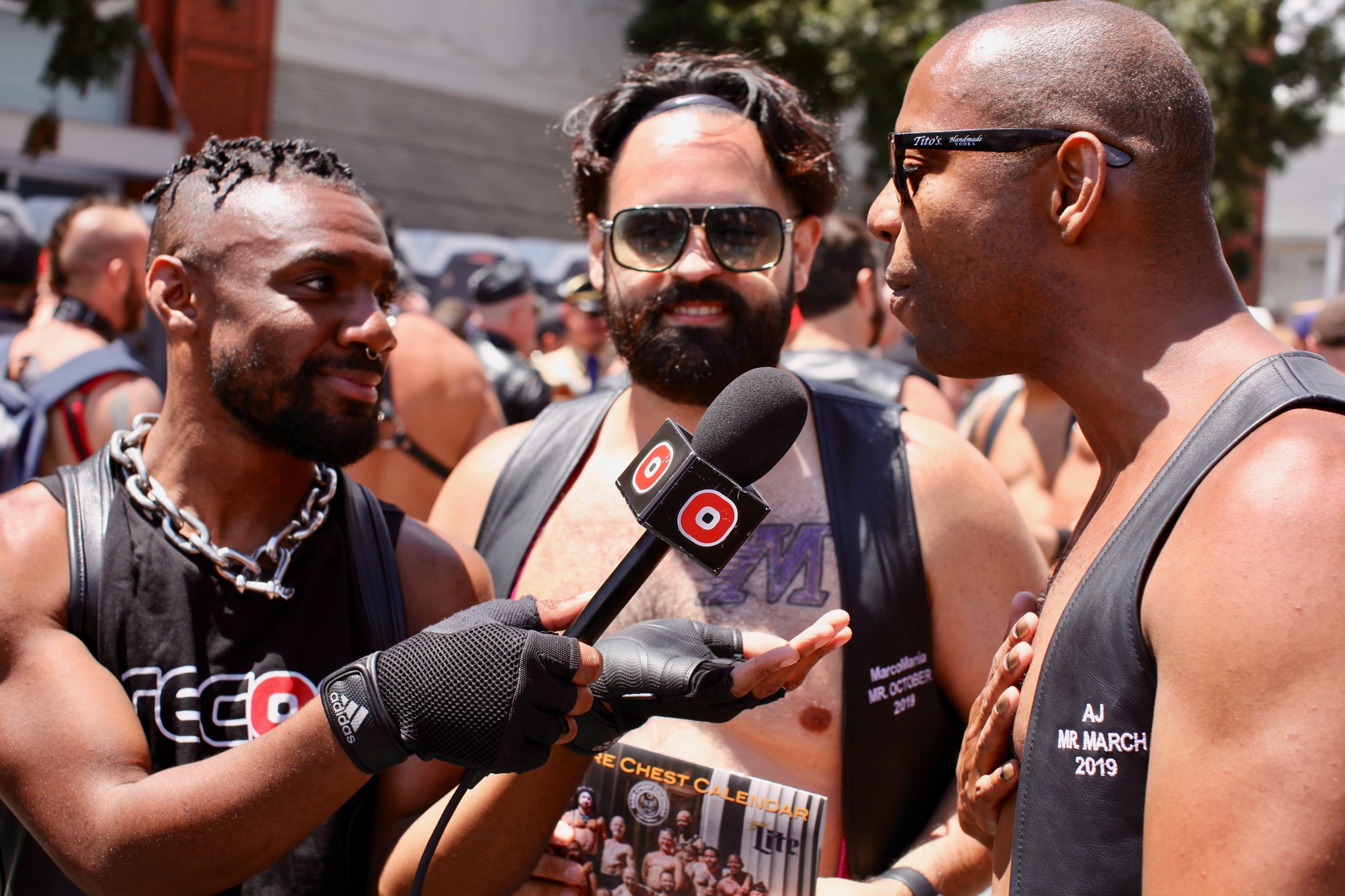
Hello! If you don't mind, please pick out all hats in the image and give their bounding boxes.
[751,881,768,893]
[556,270,608,314]
[466,257,531,305]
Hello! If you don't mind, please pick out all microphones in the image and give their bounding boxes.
[561,366,809,647]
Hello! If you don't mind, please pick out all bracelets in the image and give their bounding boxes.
[867,868,942,896]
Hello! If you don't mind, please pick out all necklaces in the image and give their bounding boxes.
[55,295,115,341]
[110,412,337,599]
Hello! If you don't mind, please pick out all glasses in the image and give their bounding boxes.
[888,129,1133,184]
[596,202,797,272]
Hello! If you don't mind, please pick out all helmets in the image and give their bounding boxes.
[575,786,597,798]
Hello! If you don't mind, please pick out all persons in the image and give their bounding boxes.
[373,50,1052,896]
[0,193,1345,526]
[557,786,769,896]
[865,0,1345,896]
[0,136,852,896]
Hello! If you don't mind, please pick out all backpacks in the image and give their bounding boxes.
[0,339,149,496]
